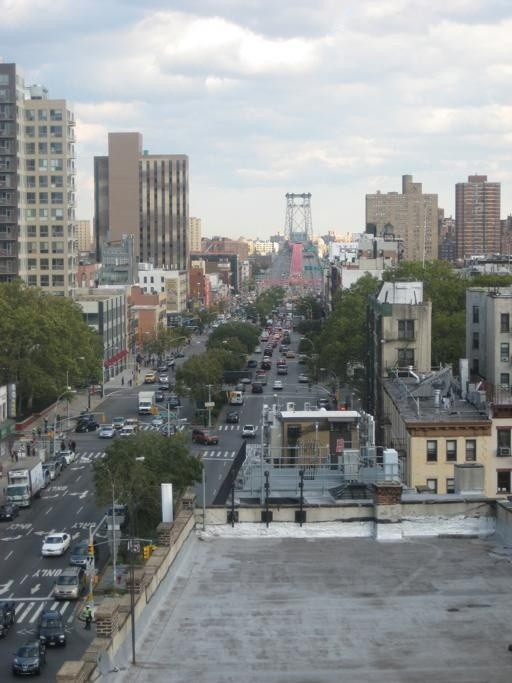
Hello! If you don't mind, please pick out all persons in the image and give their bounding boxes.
[121,354,155,386]
[37,424,42,437]
[32,424,36,439]
[82,604,92,629]
[44,414,49,427]
[61,439,65,450]
[68,438,76,452]
